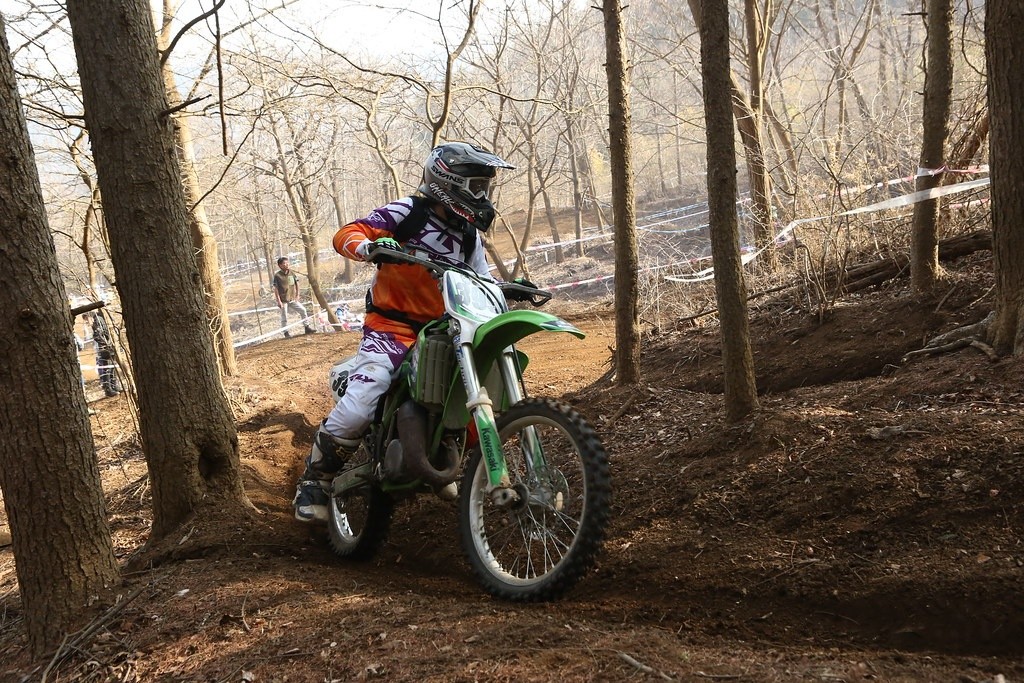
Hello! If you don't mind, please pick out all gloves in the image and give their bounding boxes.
[374,237,403,265]
[511,278,538,302]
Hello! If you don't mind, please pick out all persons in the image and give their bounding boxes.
[71,309,124,416]
[293,137,541,527]
[274,257,317,340]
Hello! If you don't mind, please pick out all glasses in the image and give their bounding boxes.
[463,173,491,201]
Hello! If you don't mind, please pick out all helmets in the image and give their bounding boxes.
[418,143,517,232]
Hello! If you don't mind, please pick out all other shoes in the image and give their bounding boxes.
[305,325,317,334]
[284,331,291,338]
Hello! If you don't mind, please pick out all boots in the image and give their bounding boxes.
[290,418,362,527]
[432,481,458,502]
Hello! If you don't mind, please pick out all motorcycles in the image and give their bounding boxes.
[320,247,615,606]
